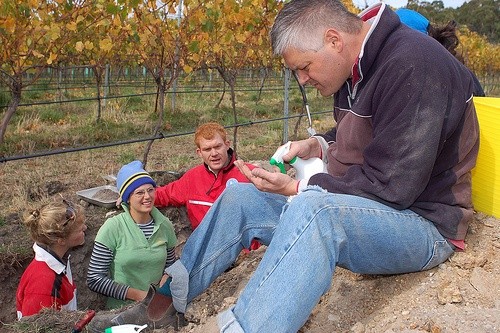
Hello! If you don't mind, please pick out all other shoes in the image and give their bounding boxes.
[89,284,190,333]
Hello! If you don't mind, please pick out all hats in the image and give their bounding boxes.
[396,8,430,36]
[116,161,156,203]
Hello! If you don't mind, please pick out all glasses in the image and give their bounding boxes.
[61,199,76,226]
[133,187,154,195]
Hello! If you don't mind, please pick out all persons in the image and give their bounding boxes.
[87,160,176,311]
[15,200,87,322]
[116,122,263,255]
[394,8,484,98]
[85,0,484,333]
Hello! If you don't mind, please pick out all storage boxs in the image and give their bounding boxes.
[471,96,500,221]
